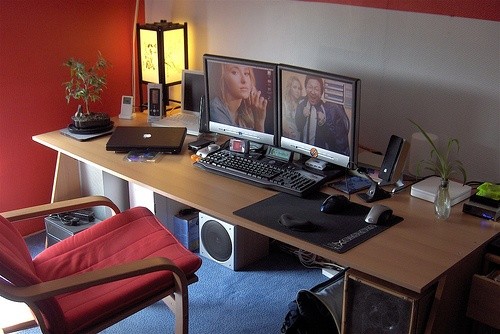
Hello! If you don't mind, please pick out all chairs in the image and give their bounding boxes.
[0,196,202,334]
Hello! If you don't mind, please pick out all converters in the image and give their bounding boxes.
[321,268,339,278]
[69,209,96,221]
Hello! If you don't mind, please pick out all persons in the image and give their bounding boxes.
[295,76,331,149]
[283,77,302,141]
[209,62,268,133]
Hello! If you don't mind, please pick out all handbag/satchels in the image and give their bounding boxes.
[279,267,350,334]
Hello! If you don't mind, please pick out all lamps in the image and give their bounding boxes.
[136,19,188,117]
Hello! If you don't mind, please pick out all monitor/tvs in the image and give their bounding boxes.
[278,63,361,179]
[203,54,277,159]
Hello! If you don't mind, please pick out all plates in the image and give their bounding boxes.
[67,121,114,134]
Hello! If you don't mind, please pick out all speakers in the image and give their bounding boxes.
[378,134,412,194]
[198,211,270,271]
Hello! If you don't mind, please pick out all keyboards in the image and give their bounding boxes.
[193,149,326,198]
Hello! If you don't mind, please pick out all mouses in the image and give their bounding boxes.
[364,204,393,224]
[319,194,350,213]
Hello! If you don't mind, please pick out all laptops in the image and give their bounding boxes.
[151,69,204,136]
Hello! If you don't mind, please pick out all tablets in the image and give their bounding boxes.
[106,125,187,154]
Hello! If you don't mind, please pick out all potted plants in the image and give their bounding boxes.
[61,47,114,134]
[406,117,467,220]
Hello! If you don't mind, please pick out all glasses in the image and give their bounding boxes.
[127,151,161,163]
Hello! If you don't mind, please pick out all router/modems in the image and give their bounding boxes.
[305,158,328,170]
[409,175,473,209]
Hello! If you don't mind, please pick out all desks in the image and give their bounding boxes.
[32,105,500,333]
